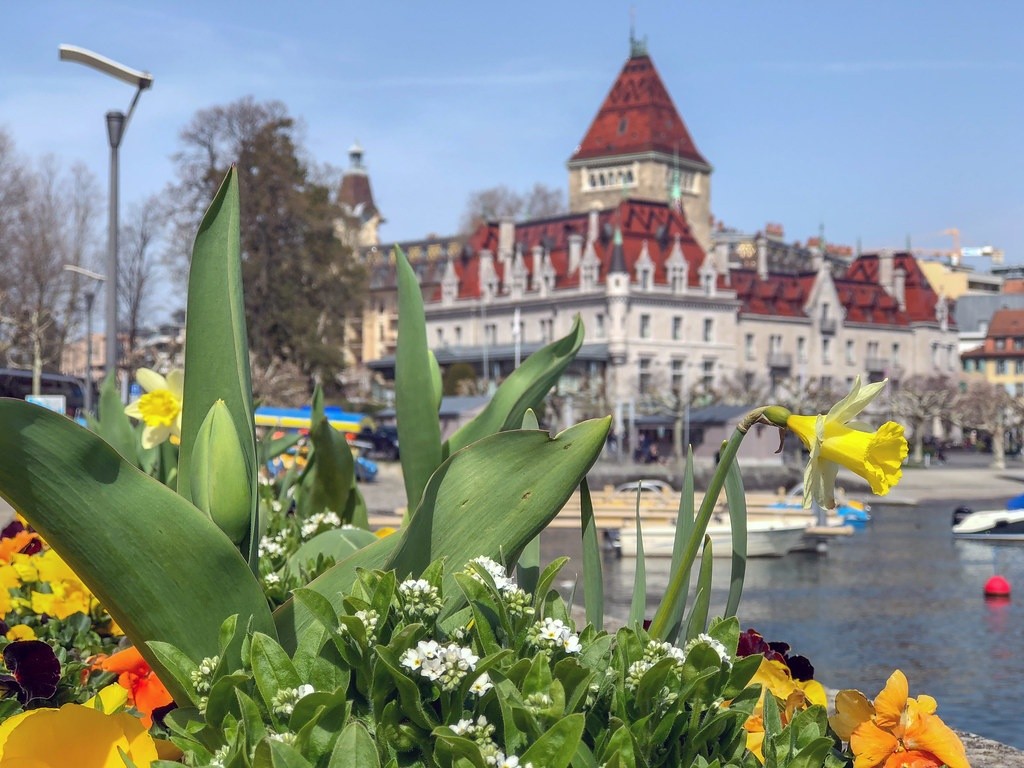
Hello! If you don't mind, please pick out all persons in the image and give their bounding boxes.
[645,442,660,465]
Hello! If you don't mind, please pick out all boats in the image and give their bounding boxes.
[949,506,1024,535]
[541,479,871,561]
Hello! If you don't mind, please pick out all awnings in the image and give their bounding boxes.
[364,341,613,370]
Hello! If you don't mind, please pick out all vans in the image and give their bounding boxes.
[0,368,98,422]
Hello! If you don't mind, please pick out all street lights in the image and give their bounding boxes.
[61,262,108,423]
[56,41,155,386]
